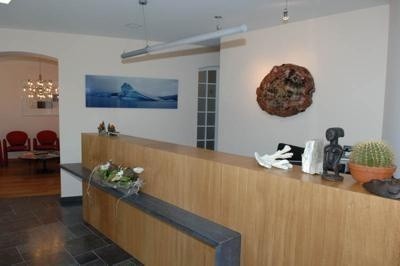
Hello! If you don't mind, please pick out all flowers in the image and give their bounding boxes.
[99,161,144,187]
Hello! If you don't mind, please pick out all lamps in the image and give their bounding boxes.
[121,0,246,60]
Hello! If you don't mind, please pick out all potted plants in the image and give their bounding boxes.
[347,141,397,184]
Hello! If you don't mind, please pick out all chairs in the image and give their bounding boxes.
[2,130,60,167]
